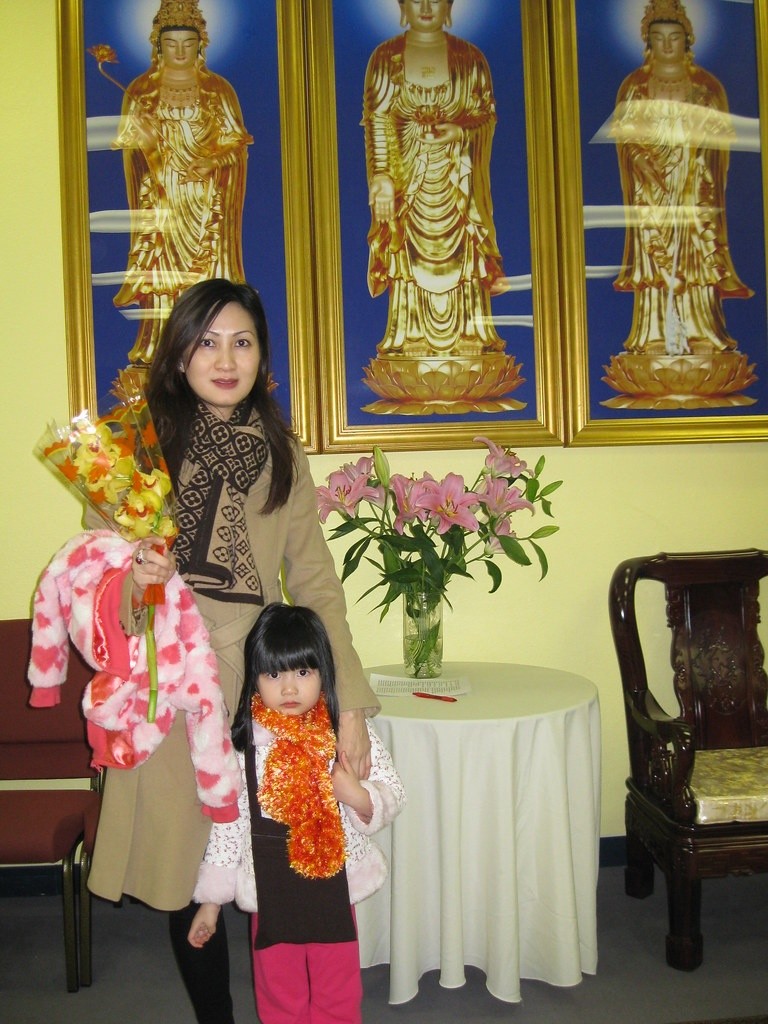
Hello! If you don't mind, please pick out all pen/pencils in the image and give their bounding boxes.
[413,692,457,703]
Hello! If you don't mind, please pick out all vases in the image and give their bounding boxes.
[402,592,444,679]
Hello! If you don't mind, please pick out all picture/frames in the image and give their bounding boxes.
[548,0,768,448]
[55,0,321,456]
[303,0,565,454]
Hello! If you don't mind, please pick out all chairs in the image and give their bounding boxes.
[0,619,108,997]
[609,548,768,971]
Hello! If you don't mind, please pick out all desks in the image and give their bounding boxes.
[355,662,601,1004]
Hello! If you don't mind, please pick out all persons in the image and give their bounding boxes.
[188,603,407,1023]
[607,0,756,355]
[83,278,381,1024]
[360,0,510,357]
[111,0,254,362]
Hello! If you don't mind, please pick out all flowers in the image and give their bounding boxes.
[35,366,193,723]
[316,437,563,623]
